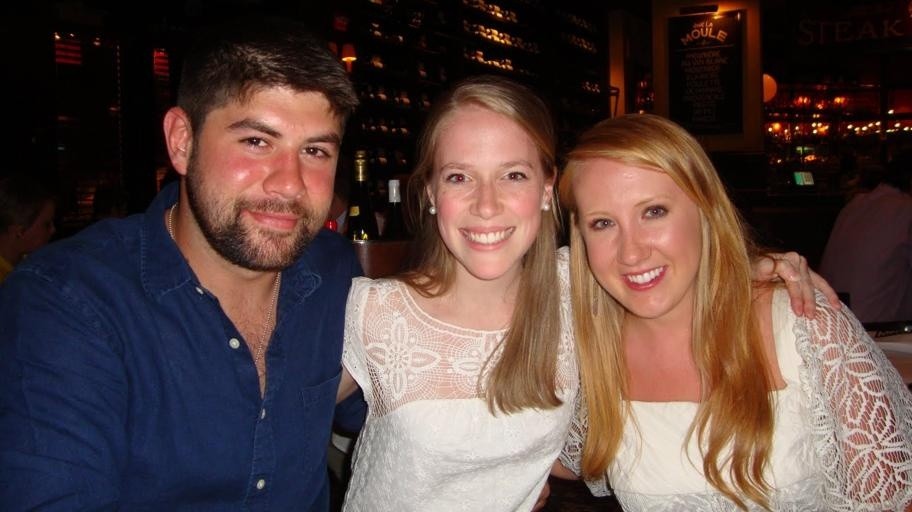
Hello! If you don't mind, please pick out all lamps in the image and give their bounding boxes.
[341,44,357,72]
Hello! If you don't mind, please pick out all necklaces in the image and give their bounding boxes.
[168,202,280,366]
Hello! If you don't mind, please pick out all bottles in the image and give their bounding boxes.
[342,151,411,240]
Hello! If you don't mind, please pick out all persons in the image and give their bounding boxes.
[1,35,356,512]
[783,133,910,322]
[1,178,136,283]
[555,111,912,512]
[340,82,843,511]
